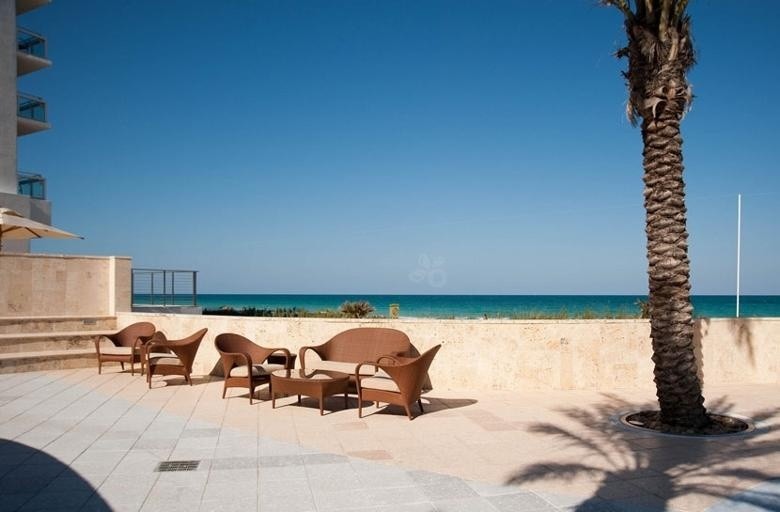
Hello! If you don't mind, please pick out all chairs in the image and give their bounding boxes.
[355,344,441,420]
[214,333,291,406]
[95,321,155,376]
[146,328,207,389]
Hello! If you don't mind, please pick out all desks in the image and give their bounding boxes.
[271,368,350,416]
[269,353,297,370]
[140,344,170,376]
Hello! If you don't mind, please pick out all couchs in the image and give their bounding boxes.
[300,327,411,409]
